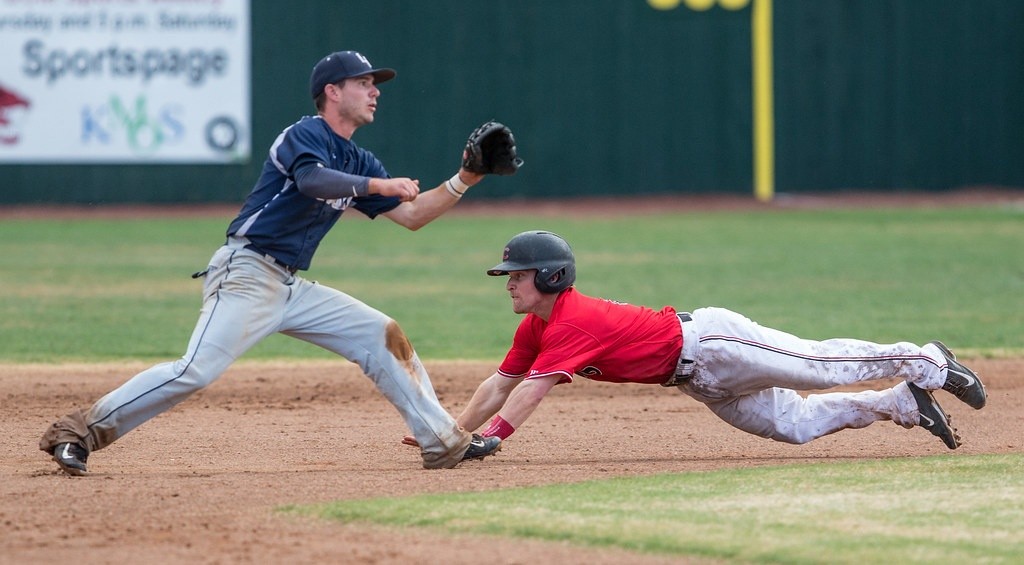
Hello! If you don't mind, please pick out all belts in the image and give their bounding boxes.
[662,314,693,387]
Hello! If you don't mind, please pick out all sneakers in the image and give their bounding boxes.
[55,443,89,476]
[906,379,962,450]
[463,434,502,461]
[931,340,988,410]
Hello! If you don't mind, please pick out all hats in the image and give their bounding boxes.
[310,50,397,99]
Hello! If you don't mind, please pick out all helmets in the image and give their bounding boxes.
[487,230,576,293]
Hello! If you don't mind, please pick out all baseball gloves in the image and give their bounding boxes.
[461,121,524,177]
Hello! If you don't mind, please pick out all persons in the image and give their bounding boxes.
[40,51,522,477]
[402,231,987,450]
